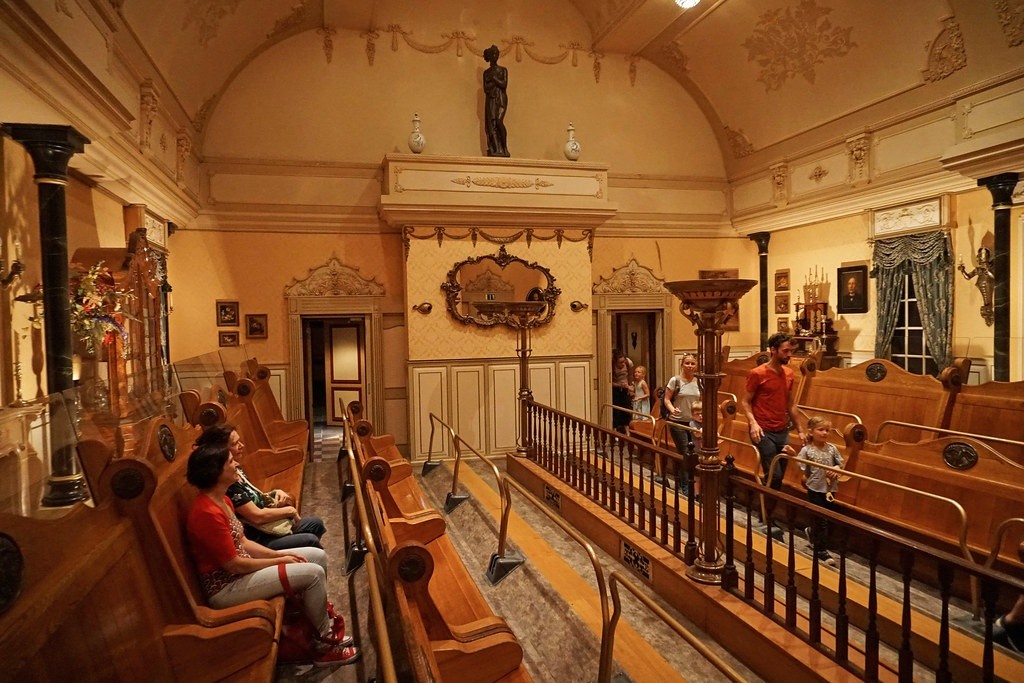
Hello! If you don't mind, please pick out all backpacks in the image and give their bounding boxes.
[656,376,680,420]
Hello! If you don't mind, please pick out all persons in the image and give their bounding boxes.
[612,351,650,449]
[664,352,700,498]
[187,445,361,666]
[192,424,327,550]
[782,416,845,565]
[742,332,808,537]
[689,401,724,455]
[843,276,861,308]
[483,45,510,157]
[959,247,995,326]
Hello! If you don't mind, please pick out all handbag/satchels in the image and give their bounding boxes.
[247,493,293,536]
[277,562,345,665]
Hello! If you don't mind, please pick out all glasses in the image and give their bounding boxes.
[684,352,695,356]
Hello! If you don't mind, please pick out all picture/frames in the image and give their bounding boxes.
[774,272,790,290]
[245,314,268,339]
[836,265,869,313]
[216,301,239,327]
[219,331,239,347]
[775,295,789,314]
[777,318,789,334]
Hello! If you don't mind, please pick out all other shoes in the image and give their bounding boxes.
[680,480,688,496]
[758,520,784,536]
[313,628,361,667]
[805,527,814,549]
[817,550,836,566]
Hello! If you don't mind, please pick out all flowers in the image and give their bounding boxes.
[63,259,140,353]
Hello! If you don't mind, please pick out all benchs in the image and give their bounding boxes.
[628,352,1024,615]
[0,356,530,683]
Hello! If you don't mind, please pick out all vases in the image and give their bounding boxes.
[408,113,426,153]
[564,121,581,160]
[80,360,107,413]
